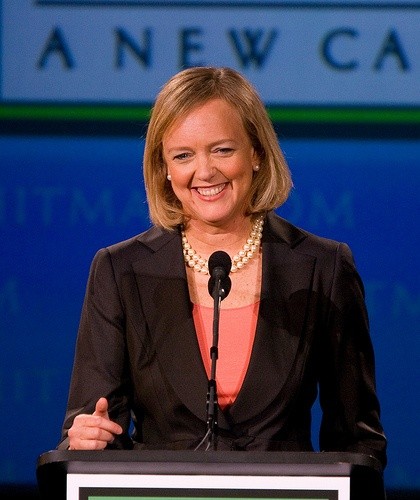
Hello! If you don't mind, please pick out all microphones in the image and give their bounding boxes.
[208,250,231,450]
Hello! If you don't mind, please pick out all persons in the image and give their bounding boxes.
[59,68,389,500]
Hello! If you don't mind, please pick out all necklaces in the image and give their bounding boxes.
[180,207,263,273]
